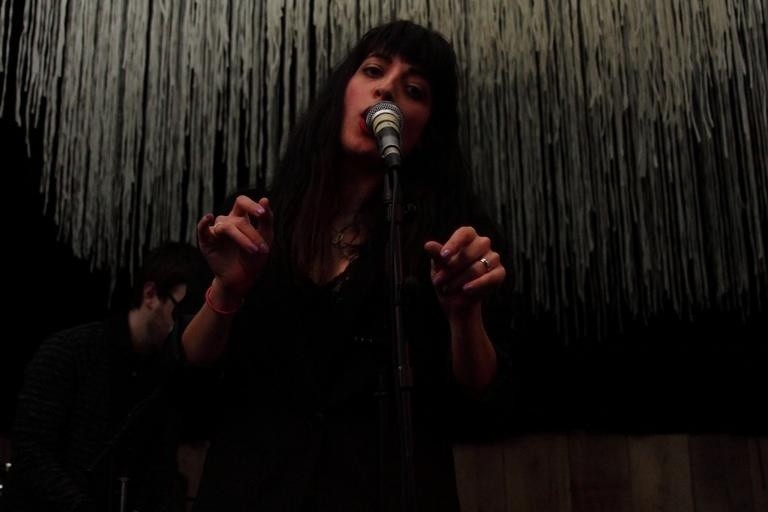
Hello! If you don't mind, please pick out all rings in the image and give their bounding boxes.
[479,256,492,273]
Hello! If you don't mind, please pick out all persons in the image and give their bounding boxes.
[0,235,217,509]
[156,18,519,510]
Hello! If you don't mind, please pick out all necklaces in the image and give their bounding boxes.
[323,196,365,248]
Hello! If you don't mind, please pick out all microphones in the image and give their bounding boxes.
[365,100,406,171]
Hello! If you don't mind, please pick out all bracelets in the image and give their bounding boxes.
[203,287,241,318]
